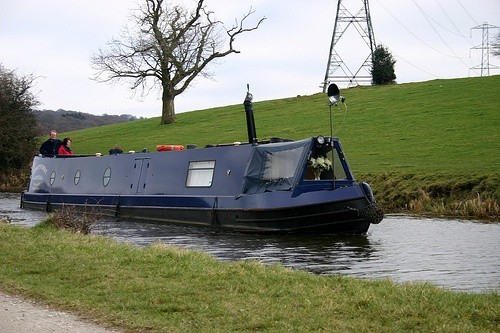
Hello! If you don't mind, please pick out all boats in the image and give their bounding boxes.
[20,83,385,242]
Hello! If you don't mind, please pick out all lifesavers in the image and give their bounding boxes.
[156,144,186,152]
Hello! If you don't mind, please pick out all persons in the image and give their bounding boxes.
[40,129,63,158]
[58,137,75,158]
[109,144,124,154]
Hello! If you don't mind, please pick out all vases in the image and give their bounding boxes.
[312,168,320,181]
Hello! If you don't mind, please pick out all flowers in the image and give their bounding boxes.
[306,155,331,176]
